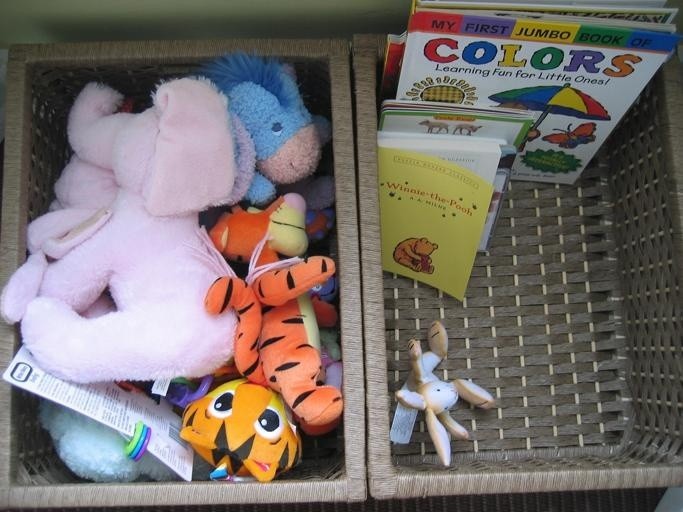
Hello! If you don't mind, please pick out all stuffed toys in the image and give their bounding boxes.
[1,54,343,484]
[0,53,344,484]
[392,320,496,467]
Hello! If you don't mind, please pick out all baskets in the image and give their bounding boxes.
[351,30,683,501]
[0,37,367,511]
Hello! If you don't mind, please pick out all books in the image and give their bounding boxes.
[375,1,678,306]
